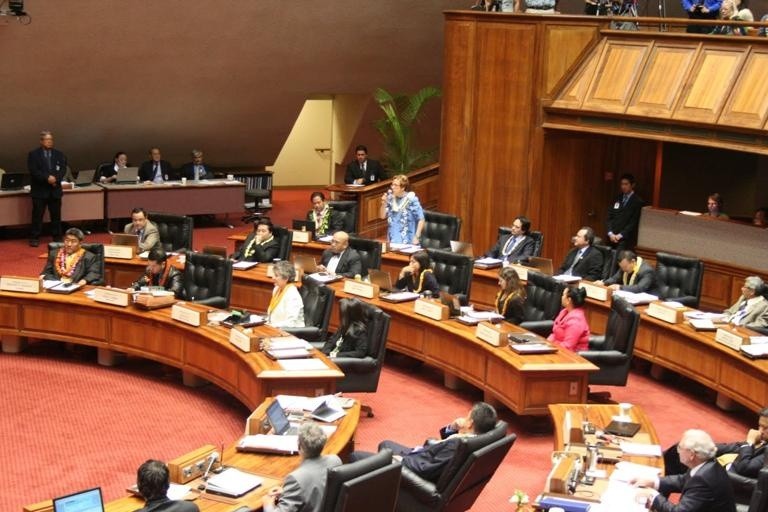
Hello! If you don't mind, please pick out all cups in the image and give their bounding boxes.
[181,178,187,184]
[617,402,634,418]
[273,258,281,269]
[423,290,432,299]
[71,182,74,189]
[227,175,233,181]
[585,444,604,471]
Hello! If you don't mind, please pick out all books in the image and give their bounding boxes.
[261,337,312,361]
[592,459,657,512]
[164,465,261,499]
[273,390,354,422]
[237,428,299,456]
[605,418,641,437]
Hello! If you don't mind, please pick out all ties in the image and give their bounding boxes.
[361,162,365,178]
[506,238,516,253]
[46,148,52,172]
[194,165,199,181]
[151,163,158,182]
[737,301,746,312]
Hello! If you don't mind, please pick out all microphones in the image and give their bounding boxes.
[381,189,392,201]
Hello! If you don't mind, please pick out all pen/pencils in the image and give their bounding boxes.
[303,409,311,414]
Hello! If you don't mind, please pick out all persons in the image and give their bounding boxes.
[378,174,425,244]
[231,219,279,264]
[343,145,391,187]
[61,163,76,182]
[306,190,343,239]
[42,228,100,285]
[719,274,768,328]
[349,402,498,472]
[467,0,767,38]
[180,149,215,180]
[28,128,66,247]
[139,148,176,182]
[605,174,643,249]
[704,192,733,223]
[128,457,202,510]
[262,424,344,511]
[100,152,139,184]
[625,430,738,512]
[123,207,161,253]
[751,207,766,226]
[318,216,658,368]
[266,259,304,328]
[665,407,767,478]
[134,249,185,300]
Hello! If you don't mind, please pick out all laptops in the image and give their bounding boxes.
[203,245,240,264]
[53,487,104,512]
[0,167,138,190]
[368,269,406,292]
[450,240,480,260]
[111,234,145,254]
[292,219,325,241]
[295,255,321,273]
[265,400,299,436]
[528,256,559,276]
[603,420,641,437]
[510,333,541,343]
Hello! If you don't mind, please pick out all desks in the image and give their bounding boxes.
[224,211,766,421]
[34,231,601,424]
[0,269,348,412]
[10,392,362,512]
[521,398,671,512]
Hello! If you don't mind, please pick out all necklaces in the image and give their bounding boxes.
[55,247,86,275]
[267,285,290,314]
[244,235,273,257]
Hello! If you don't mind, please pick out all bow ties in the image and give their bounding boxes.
[332,251,340,257]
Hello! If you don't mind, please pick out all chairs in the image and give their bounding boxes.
[2,164,273,238]
[568,236,624,285]
[254,272,337,340]
[386,415,517,509]
[405,245,477,311]
[322,234,385,285]
[482,265,572,346]
[38,241,108,289]
[314,446,407,509]
[307,294,393,425]
[482,223,543,263]
[638,248,707,312]
[166,249,235,310]
[229,221,296,268]
[407,206,463,255]
[557,290,643,407]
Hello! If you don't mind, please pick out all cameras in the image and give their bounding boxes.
[694,5,703,14]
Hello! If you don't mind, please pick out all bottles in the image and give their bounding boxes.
[502,256,509,268]
